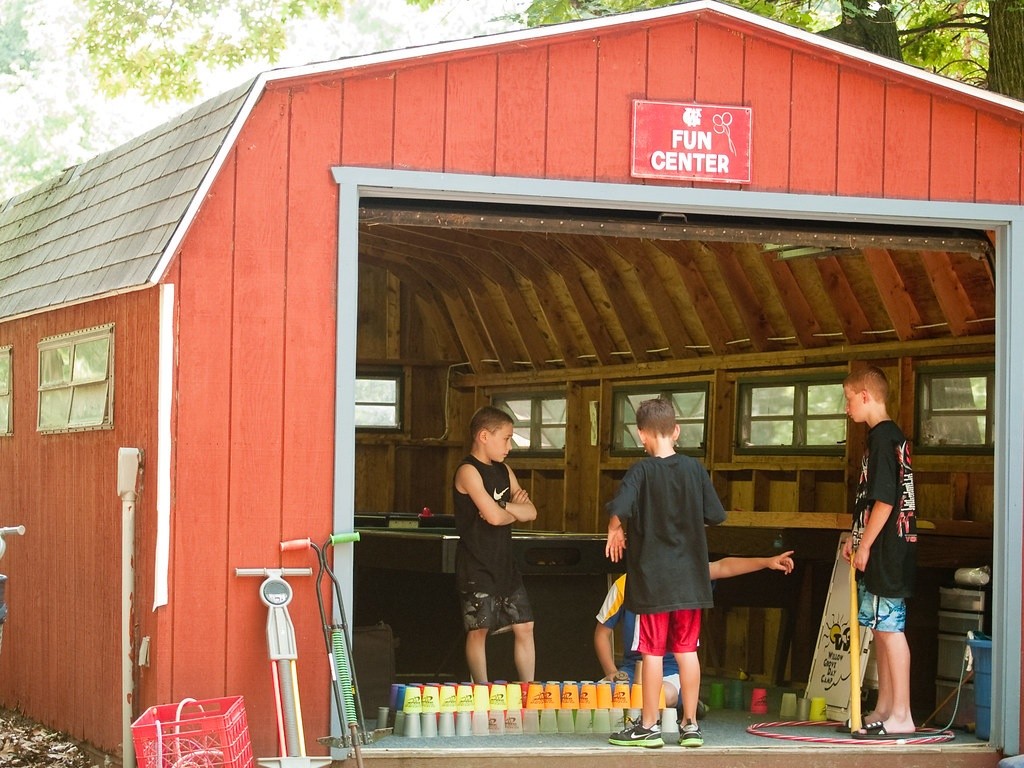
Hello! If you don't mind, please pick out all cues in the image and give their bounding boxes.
[920,670,974,729]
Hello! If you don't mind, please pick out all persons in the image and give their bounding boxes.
[604,399,728,747]
[452,406,538,684]
[594,550,794,719]
[836,365,917,740]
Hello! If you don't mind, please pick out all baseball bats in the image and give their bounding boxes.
[847,549,868,735]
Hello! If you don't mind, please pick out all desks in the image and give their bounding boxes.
[358,528,845,684]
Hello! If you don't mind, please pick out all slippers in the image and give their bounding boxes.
[852,721,917,739]
[836,714,867,733]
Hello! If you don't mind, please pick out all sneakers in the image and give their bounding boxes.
[608,715,664,747]
[678,719,704,746]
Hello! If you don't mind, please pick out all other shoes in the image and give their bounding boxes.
[681,699,709,720]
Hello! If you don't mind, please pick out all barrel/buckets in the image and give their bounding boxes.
[966,630,992,740]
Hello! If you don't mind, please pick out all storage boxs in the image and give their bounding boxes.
[934,588,993,743]
[131,696,257,768]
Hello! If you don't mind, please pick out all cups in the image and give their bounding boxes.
[750,688,768,714]
[780,694,796,721]
[796,698,811,722]
[726,679,744,710]
[809,697,828,721]
[709,683,725,709]
[375,661,681,738]
